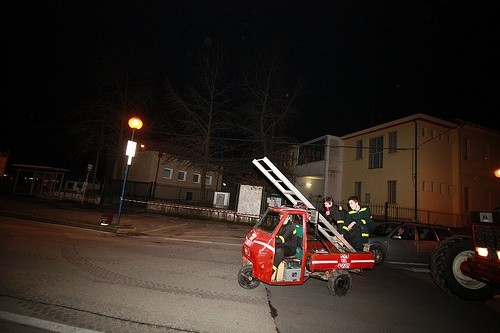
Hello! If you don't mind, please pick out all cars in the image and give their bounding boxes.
[362,221,457,266]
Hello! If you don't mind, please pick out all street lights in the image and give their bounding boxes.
[113,116,142,228]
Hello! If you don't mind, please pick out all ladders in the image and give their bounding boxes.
[252,157,357,253]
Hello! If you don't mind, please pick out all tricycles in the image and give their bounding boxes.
[237,206,375,297]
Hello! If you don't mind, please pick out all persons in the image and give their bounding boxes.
[331,196,373,251]
[318,197,348,240]
[272,213,297,275]
[397,228,408,239]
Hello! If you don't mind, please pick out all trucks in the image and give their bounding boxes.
[430,207,500,302]
